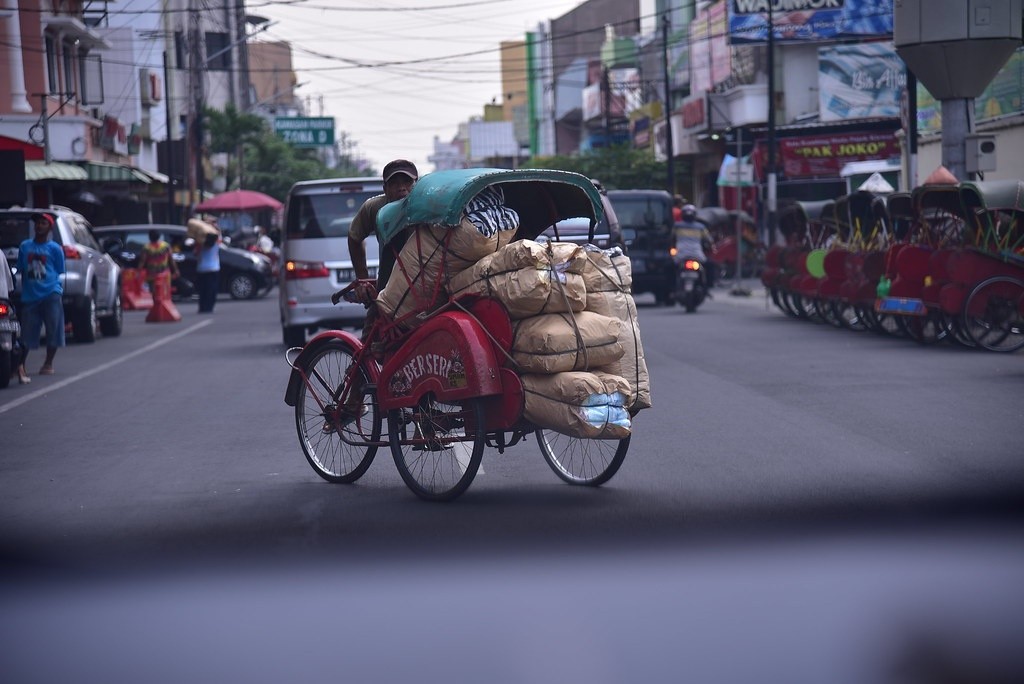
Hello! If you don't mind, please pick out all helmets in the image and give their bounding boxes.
[681,204,696,221]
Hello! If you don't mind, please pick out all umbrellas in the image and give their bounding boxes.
[196,188,283,246]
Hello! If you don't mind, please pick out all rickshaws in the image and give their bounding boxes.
[762,176,1024,358]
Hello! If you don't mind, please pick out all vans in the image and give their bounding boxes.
[277,175,386,351]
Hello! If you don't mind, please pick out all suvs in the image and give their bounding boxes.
[0,201,127,345]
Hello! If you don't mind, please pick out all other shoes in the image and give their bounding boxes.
[20,376,32,385]
[39,367,54,375]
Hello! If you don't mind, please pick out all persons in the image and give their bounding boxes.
[0,250,30,384]
[193,234,222,312]
[19,213,66,375]
[323,160,453,452]
[135,230,180,302]
[670,204,718,289]
[254,225,273,254]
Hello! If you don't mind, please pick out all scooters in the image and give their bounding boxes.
[667,244,720,313]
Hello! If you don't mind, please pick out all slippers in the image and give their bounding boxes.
[321,405,369,433]
[412,435,454,450]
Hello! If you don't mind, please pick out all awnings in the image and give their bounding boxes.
[25,160,87,180]
[84,163,153,184]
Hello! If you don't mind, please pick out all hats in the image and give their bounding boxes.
[31,212,54,226]
[383,159,418,183]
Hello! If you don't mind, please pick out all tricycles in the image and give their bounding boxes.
[285,168,644,504]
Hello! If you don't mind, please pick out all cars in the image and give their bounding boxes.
[0,248,23,390]
[92,223,275,301]
[534,185,761,314]
